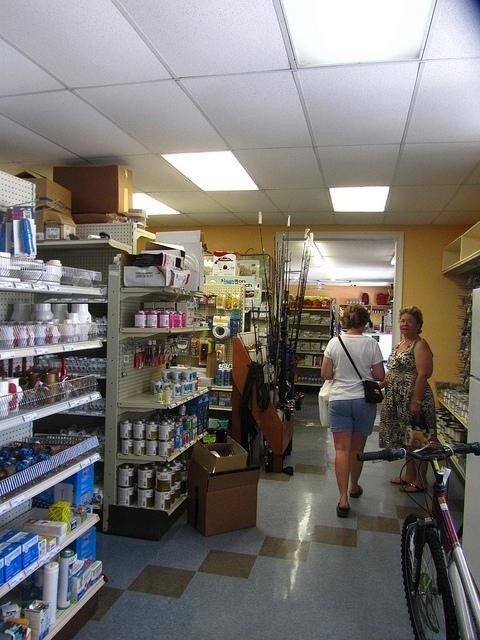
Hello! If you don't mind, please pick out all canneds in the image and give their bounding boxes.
[434,387,469,443]
[118,307,198,512]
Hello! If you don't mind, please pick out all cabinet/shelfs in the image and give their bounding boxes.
[282,296,335,387]
[34,222,212,543]
[175,252,271,465]
[338,304,391,333]
[441,221,480,276]
[431,393,469,481]
[0,170,108,640]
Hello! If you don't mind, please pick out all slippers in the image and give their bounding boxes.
[399,483,427,493]
[391,476,411,485]
[350,485,363,498]
[338,502,351,518]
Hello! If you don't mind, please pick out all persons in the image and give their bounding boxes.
[320,302,388,519]
[379,307,437,495]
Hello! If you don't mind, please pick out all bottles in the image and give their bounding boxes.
[10,300,93,342]
[134,309,188,327]
[42,562,62,627]
[56,549,75,609]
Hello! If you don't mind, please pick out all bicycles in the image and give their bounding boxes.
[357,441,480,640]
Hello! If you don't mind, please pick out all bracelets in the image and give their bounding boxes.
[411,397,423,405]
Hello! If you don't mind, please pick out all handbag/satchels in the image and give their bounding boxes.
[363,380,384,404]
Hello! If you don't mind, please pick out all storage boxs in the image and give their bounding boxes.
[15,170,72,216]
[187,460,261,537]
[191,435,249,476]
[52,165,134,214]
[71,213,127,225]
